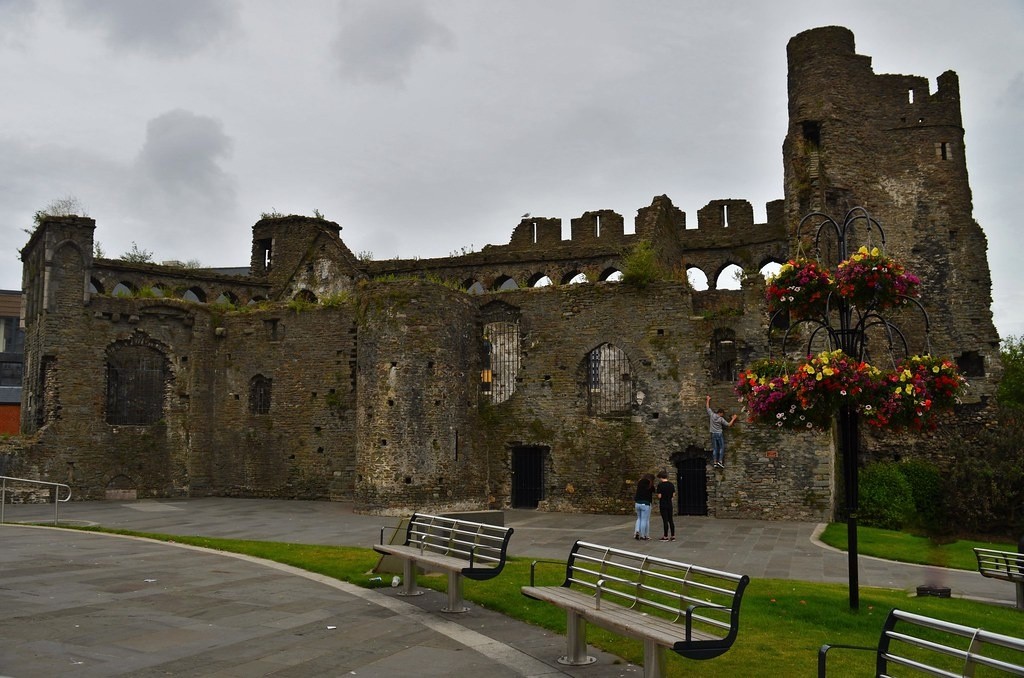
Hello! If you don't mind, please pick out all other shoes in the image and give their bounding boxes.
[633,532,652,541]
[659,536,669,541]
[718,462,724,468]
[670,536,676,541]
[714,464,720,468]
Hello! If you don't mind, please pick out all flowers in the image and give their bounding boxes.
[764,246,924,323]
[732,347,969,434]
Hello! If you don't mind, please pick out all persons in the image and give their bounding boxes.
[633,472,654,540]
[705,395,739,469]
[655,471,677,542]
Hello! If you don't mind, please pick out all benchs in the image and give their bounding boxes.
[520,539,750,678]
[373,512,515,613]
[818,607,1024,678]
[972,548,1024,608]
